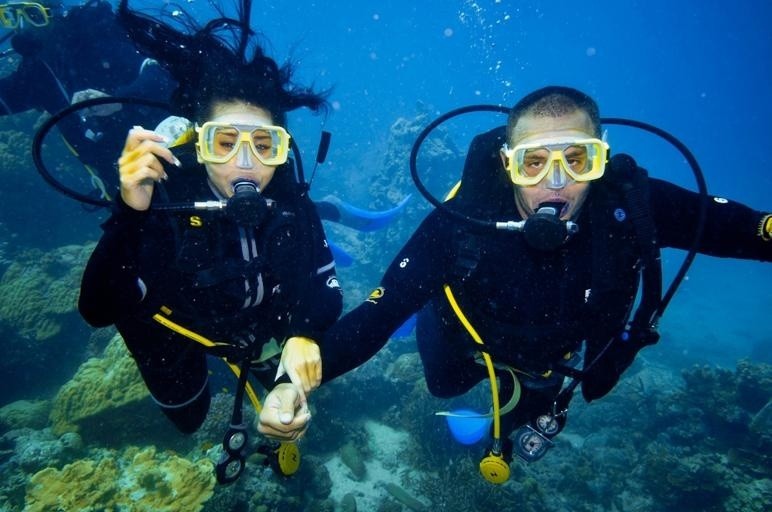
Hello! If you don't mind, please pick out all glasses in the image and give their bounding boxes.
[194,120,291,169]
[501,131,611,190]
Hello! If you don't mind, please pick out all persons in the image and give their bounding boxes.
[254,82,771,441]
[77,0,343,437]
